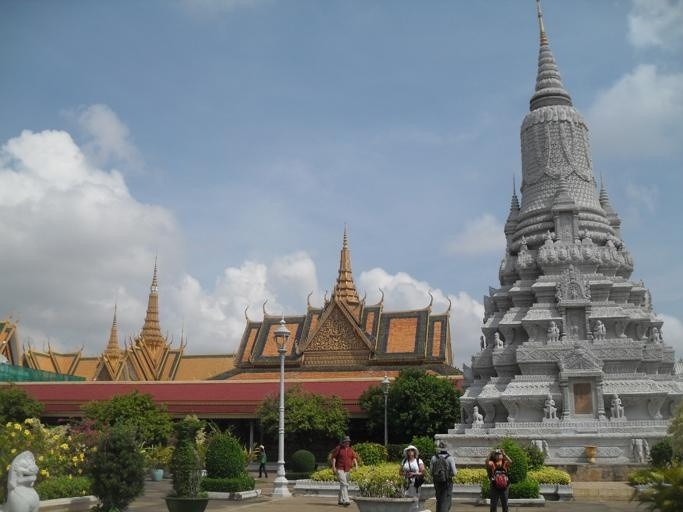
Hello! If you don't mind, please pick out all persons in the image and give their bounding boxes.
[543,229,553,241]
[398,445,426,508]
[428,441,457,511]
[484,447,513,511]
[610,393,624,418]
[546,320,559,341]
[331,435,359,506]
[582,228,591,242]
[257,444,268,477]
[519,235,528,252]
[593,319,606,341]
[542,393,557,420]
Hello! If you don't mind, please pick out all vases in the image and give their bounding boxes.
[352,493,418,512]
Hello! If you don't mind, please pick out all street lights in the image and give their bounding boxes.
[379,374,391,444]
[266,312,295,496]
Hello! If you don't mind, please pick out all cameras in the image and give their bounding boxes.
[496,448,502,453]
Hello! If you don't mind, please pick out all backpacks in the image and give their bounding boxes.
[432,453,454,490]
[491,459,510,492]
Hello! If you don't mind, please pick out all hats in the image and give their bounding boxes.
[342,436,351,443]
[436,440,449,452]
[403,445,420,458]
[260,445,265,450]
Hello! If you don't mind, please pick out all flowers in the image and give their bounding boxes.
[358,465,408,498]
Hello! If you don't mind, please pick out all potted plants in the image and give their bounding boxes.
[162,432,208,512]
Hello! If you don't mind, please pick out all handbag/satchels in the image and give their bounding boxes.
[413,474,425,489]
